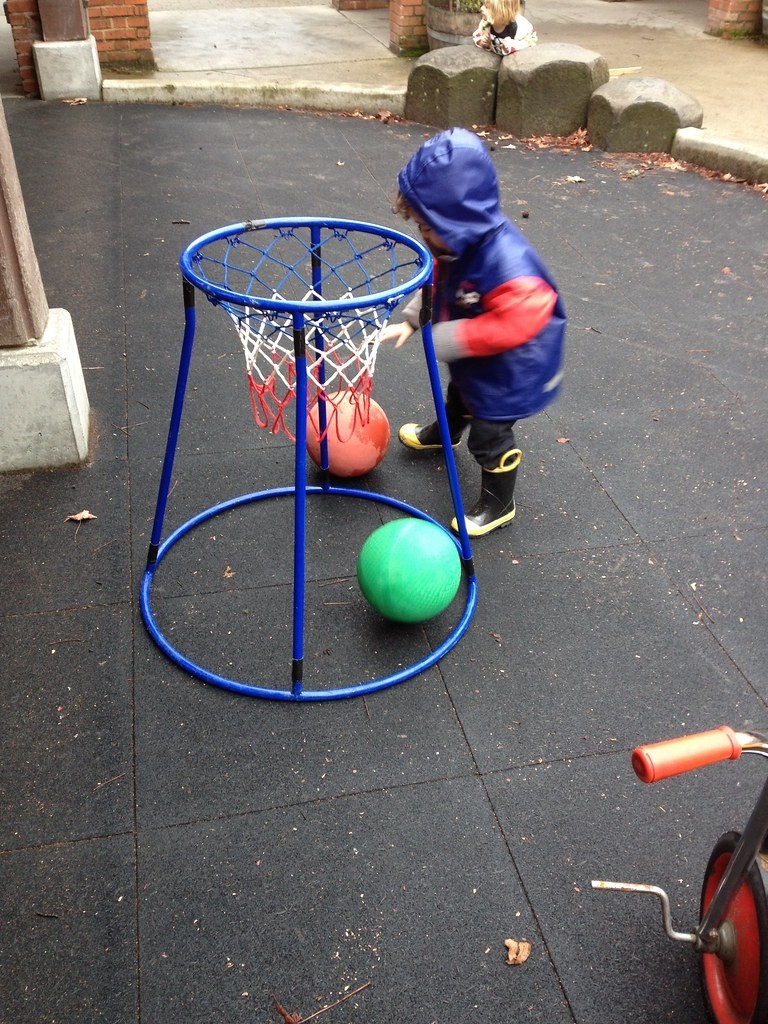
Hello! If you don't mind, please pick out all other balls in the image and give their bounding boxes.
[306,390,390,478]
[359,517,462,625]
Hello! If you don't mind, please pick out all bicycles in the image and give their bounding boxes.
[591,725,767,1024]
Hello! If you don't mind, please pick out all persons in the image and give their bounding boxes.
[366,128,568,539]
[471,0,539,55]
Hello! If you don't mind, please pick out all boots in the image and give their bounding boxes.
[397,400,474,452]
[449,448,523,540]
